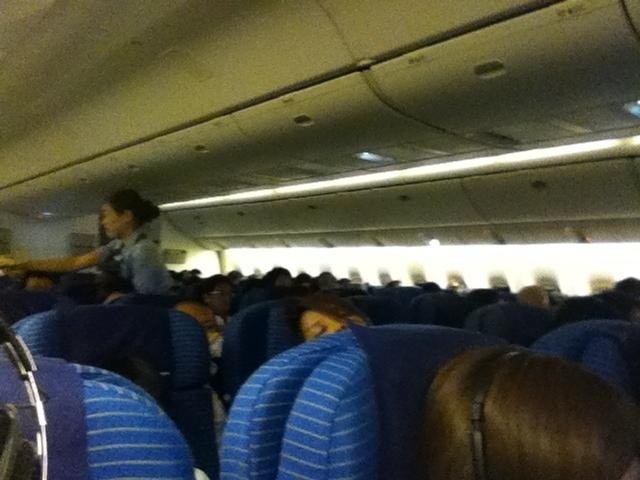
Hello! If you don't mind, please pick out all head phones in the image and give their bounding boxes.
[0,317,48,480]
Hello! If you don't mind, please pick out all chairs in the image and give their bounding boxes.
[219,327,382,480]
[0,272,639,403]
[74,362,195,480]
